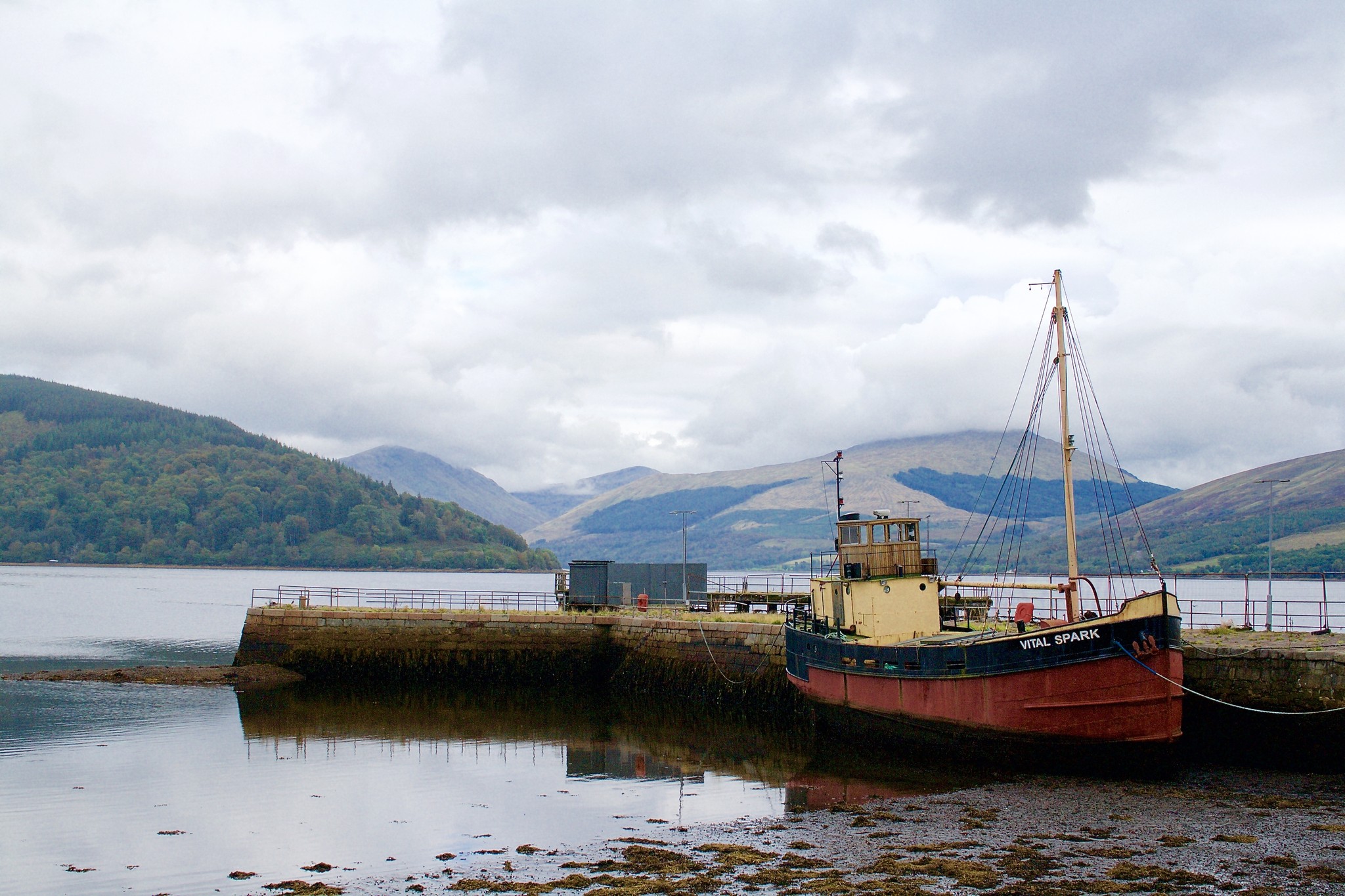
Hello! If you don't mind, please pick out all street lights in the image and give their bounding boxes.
[1252,479,1291,632]
[668,511,698,601]
[925,515,931,559]
[896,500,921,519]
[674,510,697,563]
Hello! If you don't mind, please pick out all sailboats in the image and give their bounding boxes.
[779,267,1186,749]
